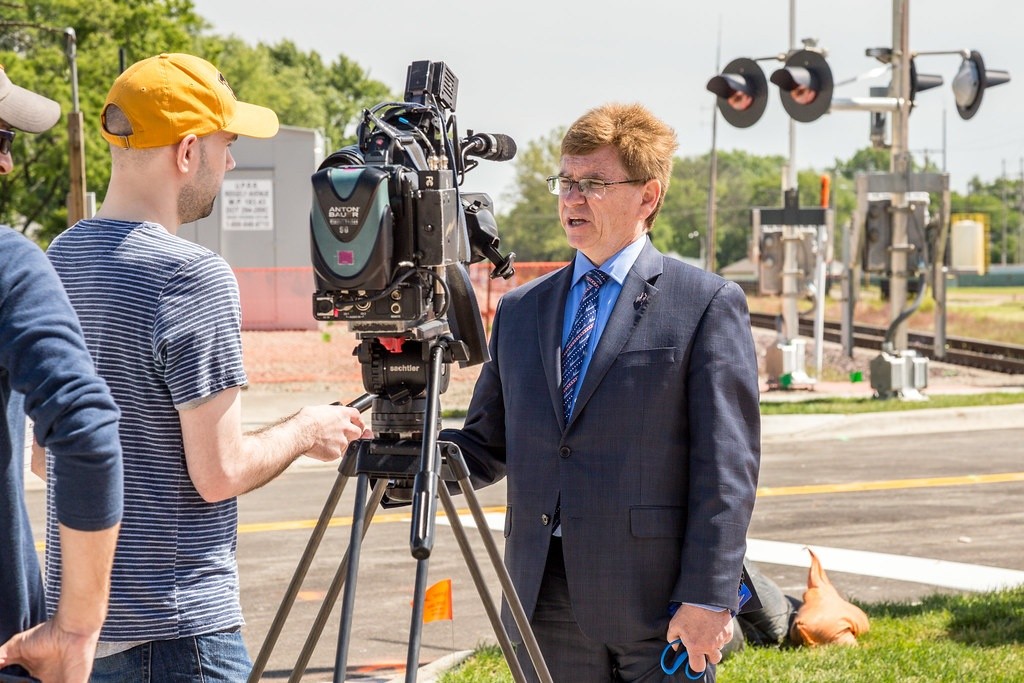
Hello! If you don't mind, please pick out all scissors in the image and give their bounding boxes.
[661,592,746,680]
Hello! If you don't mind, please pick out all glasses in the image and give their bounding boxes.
[0,129,15,155]
[546,176,648,199]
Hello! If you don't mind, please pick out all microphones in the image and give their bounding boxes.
[469,132,517,162]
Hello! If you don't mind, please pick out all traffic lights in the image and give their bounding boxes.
[951,50,1012,120]
[707,58,769,128]
[771,48,833,122]
[869,58,942,117]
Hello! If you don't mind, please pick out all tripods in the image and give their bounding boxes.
[244,318,554,683]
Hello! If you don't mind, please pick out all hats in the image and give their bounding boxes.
[0,68,61,133]
[100,53,279,150]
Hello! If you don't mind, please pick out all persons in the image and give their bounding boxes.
[31,52,368,682]
[0,64,124,682]
[361,106,761,682]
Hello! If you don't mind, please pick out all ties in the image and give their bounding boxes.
[560,268,610,426]
